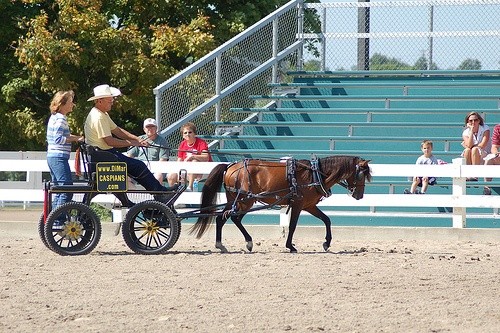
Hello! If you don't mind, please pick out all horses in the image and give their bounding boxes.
[185,153,372,252]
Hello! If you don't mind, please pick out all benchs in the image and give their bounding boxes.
[167,70,500,228]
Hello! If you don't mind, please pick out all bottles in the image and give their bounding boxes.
[193,179,198,192]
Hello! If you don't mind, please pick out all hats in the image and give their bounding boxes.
[143,118,157,127]
[87,84,120,101]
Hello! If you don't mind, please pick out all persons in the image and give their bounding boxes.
[126,118,170,185]
[46,89,83,225]
[84,84,178,202]
[483,124,500,194]
[403,140,437,194]
[166,122,213,191]
[460,111,490,188]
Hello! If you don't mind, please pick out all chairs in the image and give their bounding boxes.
[80,141,125,191]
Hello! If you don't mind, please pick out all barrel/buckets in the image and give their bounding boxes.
[111,196,129,223]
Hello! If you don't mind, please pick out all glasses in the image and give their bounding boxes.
[145,126,156,128]
[184,132,193,134]
[469,119,479,122]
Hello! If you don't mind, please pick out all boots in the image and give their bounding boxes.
[134,168,178,202]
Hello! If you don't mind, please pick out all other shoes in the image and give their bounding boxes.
[466,177,470,188]
[414,188,422,194]
[483,186,491,195]
[403,189,411,194]
[469,177,479,188]
